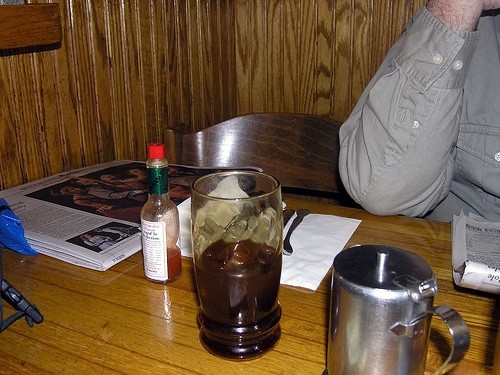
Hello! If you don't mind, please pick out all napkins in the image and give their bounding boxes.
[159,196,362,292]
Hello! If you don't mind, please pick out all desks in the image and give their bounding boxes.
[0,193,500,375]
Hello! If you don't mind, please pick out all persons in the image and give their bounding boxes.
[80,234,115,252]
[95,226,139,239]
[338,0,500,231]
[52,164,207,224]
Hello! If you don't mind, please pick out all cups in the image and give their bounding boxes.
[190,170,284,362]
[326,244,471,375]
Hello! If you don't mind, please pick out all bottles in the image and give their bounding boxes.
[140,144,183,284]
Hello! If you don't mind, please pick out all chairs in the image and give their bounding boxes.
[162,112,357,206]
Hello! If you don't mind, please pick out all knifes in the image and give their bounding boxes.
[284,208,310,252]
